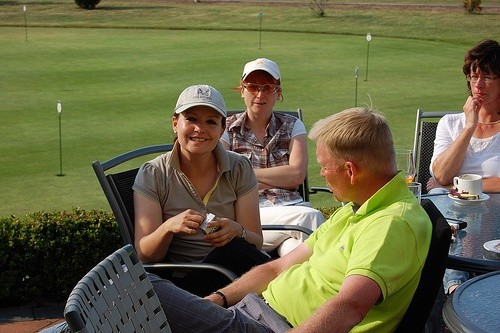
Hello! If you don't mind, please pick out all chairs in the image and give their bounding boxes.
[65,108,465,333]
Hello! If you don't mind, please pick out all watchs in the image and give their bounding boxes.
[241,225,247,239]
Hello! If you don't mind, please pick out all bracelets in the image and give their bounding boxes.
[213,291,227,307]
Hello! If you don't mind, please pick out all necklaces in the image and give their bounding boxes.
[478,120,500,124]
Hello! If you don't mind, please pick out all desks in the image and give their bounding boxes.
[423,192,500,274]
[442,271,500,333]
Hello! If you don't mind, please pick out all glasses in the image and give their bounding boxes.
[242,83,280,95]
[466,74,500,84]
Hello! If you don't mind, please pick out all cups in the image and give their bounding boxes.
[394,149,416,183]
[407,183,421,205]
[454,174,482,198]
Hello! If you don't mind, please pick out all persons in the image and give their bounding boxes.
[427,40,500,295]
[218,57,325,259]
[132,84,271,279]
[122,101,432,333]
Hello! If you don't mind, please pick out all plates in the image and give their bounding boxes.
[448,193,490,205]
[483,240,500,253]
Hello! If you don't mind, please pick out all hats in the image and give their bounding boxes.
[240,57,281,82]
[174,84,227,118]
